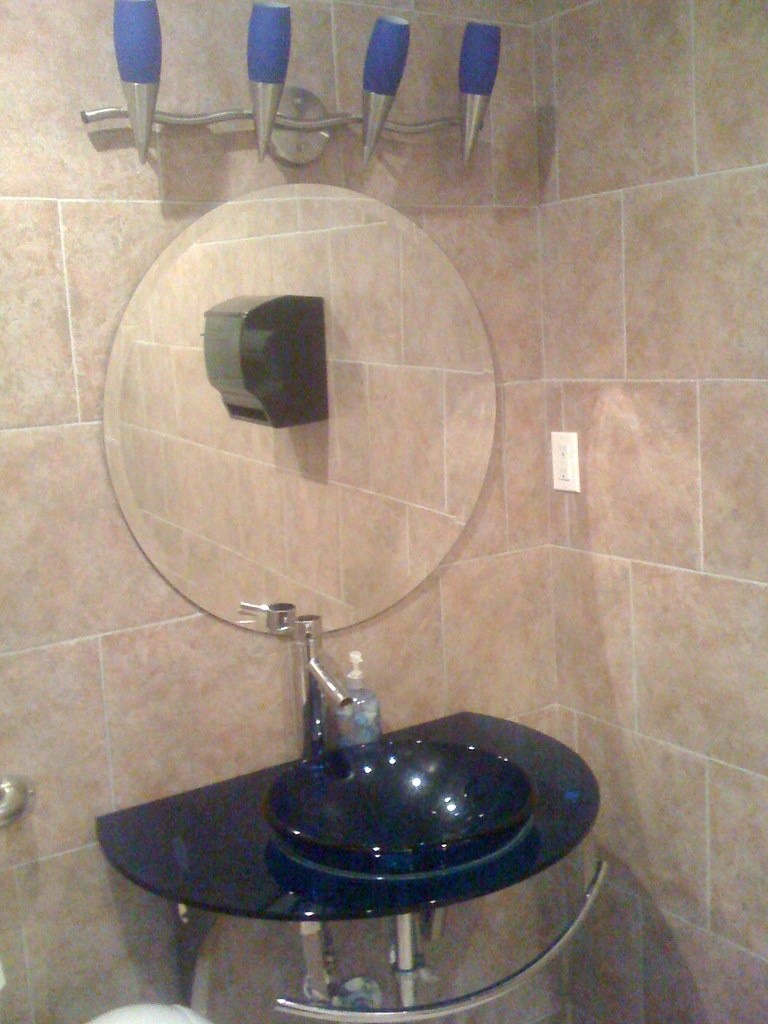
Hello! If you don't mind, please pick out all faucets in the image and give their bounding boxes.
[275,615,353,761]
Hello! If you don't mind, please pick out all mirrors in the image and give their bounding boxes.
[103,183,496,635]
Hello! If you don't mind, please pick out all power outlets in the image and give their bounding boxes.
[551,432,580,493]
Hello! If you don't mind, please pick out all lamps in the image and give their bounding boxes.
[81,0,502,167]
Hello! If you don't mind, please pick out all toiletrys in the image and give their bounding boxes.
[334,649,380,747]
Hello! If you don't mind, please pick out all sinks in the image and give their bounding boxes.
[260,739,538,887]
